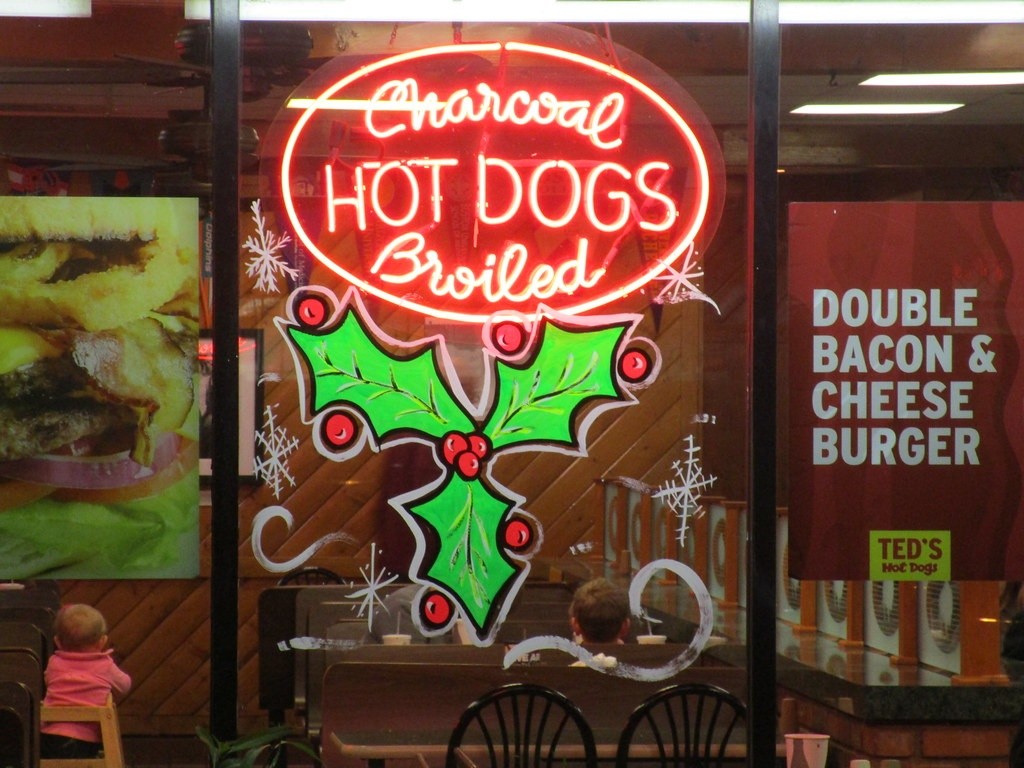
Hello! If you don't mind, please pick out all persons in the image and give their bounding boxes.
[39,604,130,760]
[569,578,632,646]
[371,582,455,646]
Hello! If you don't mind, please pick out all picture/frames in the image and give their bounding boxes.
[196,327,265,486]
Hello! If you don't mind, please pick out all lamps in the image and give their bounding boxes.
[859,26,1023,88]
[787,69,965,116]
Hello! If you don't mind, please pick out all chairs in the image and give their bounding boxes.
[445,681,597,768]
[615,681,745,768]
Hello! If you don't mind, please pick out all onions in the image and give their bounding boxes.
[0,426,185,489]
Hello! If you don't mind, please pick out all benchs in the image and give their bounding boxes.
[329,723,840,768]
[257,584,746,768]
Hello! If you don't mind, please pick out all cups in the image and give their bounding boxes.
[700,636,727,650]
[783,734,830,767]
[383,634,413,646]
[636,635,667,644]
[0,583,24,592]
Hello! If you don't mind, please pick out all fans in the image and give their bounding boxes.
[0,23,402,222]
